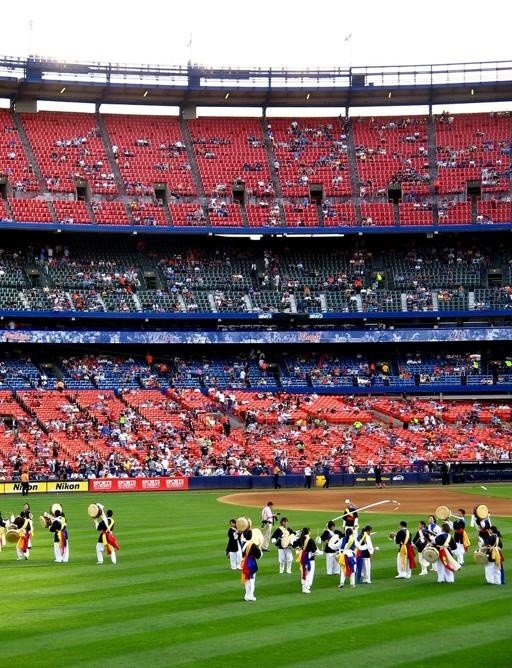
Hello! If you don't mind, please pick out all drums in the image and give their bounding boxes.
[40,514,54,528]
[436,506,450,520]
[474,546,488,564]
[235,516,251,531]
[50,504,63,515]
[6,525,19,542]
[88,504,102,518]
[328,534,342,549]
[240,528,264,547]
[281,536,296,549]
[422,543,440,563]
[475,504,489,519]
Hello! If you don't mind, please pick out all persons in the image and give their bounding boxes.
[49,510,68,562]
[0,111,510,495]
[22,503,34,548]
[60,511,68,523]
[92,509,119,565]
[9,511,30,560]
[225,499,504,600]
[0,511,7,554]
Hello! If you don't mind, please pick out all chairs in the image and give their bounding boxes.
[0,344,512,482]
[0,110,512,226]
[1,230,511,311]
